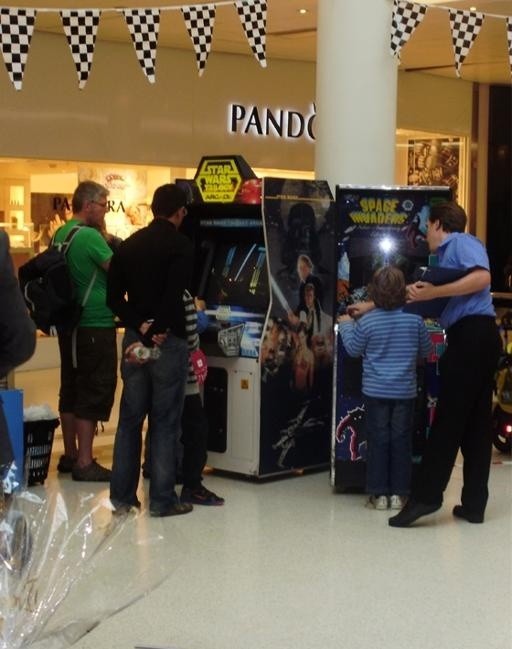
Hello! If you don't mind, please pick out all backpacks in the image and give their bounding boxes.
[21,223,98,339]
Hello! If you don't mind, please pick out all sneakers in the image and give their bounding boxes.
[370,494,406,509]
[143,465,223,517]
[109,494,141,509]
[57,454,112,482]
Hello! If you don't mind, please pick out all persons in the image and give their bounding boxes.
[346,204,502,527]
[107,184,193,516]
[1,228,36,512]
[142,290,226,504]
[49,182,124,481]
[288,283,319,346]
[335,266,433,510]
[296,254,322,305]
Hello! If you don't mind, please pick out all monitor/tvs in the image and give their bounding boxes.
[197,241,270,312]
[346,230,430,287]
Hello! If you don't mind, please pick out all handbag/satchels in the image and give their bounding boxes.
[191,350,209,385]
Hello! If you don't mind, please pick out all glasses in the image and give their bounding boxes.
[92,200,108,209]
[183,209,188,217]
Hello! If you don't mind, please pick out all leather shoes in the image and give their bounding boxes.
[453,505,483,523]
[389,500,442,527]
